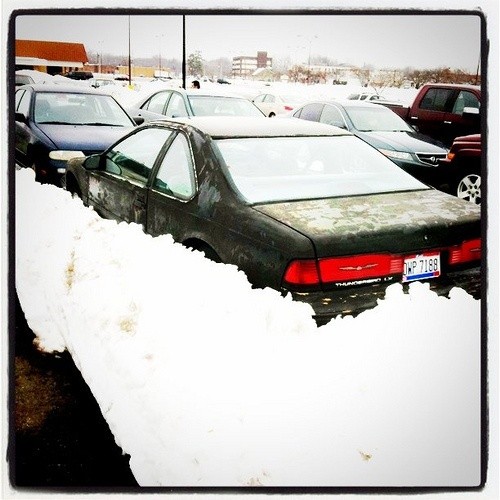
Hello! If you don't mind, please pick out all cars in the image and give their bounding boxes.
[14,69,416,116]
[128,86,267,126]
[440,132,480,204]
[294,99,450,187]
[64,114,481,327]
[13,83,145,198]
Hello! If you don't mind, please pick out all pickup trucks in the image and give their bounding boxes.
[374,82,481,138]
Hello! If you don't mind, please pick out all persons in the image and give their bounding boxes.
[191,80,200,89]
